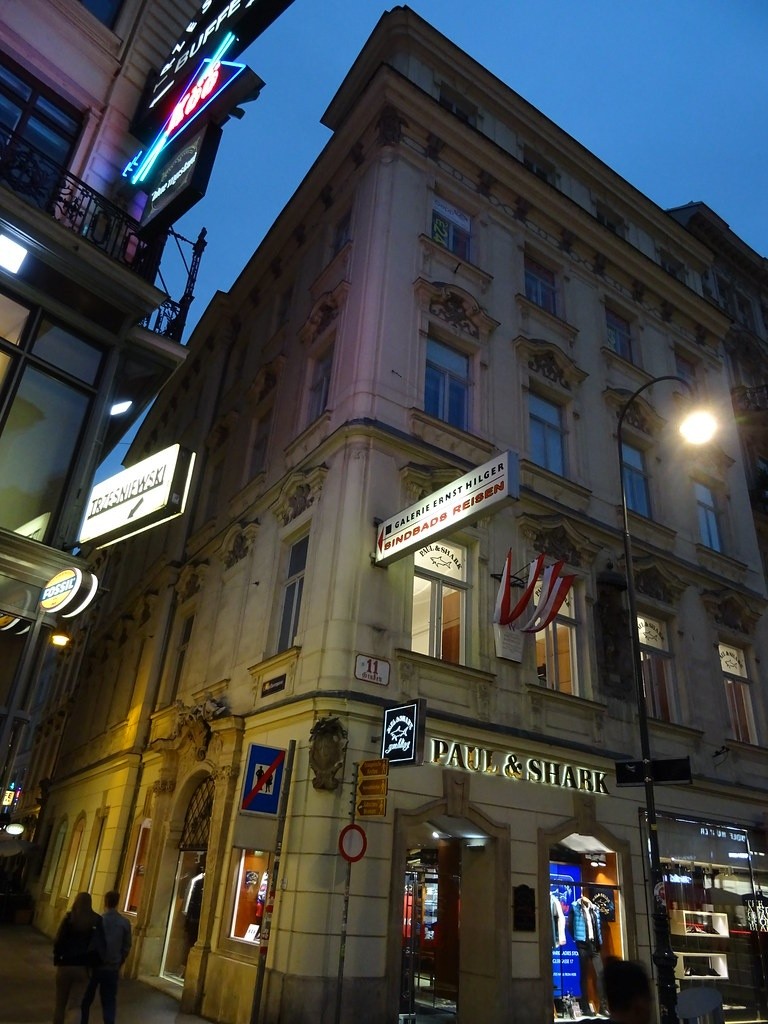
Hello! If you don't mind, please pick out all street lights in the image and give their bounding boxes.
[614,376,741,1023]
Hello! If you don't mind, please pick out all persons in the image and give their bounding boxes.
[80,891,135,1024]
[52,892,104,1023]
[577,956,654,1024]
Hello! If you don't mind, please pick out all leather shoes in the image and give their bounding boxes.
[582,1008,595,1017]
[599,1007,613,1017]
[685,966,721,976]
[686,924,720,935]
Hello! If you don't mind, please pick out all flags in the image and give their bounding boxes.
[495,548,576,634]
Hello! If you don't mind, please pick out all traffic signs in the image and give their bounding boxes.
[352,759,388,818]
